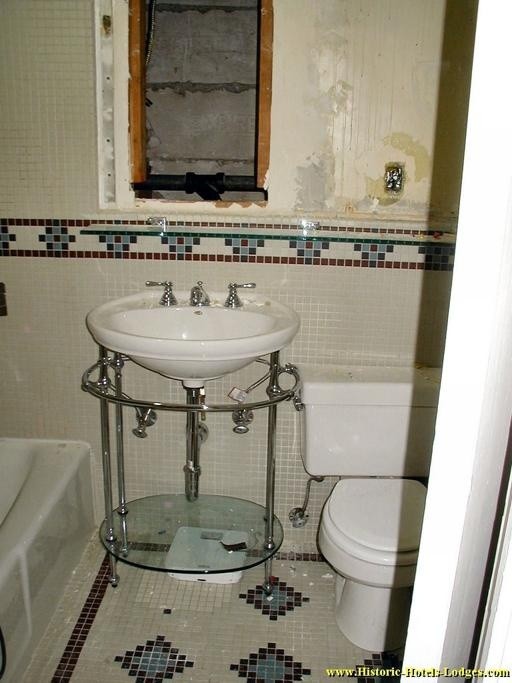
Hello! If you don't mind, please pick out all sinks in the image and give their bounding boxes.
[86,290,300,389]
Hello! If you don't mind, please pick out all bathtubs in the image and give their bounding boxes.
[0,436,96,683]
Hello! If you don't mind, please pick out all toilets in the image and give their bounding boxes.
[297,365,442,654]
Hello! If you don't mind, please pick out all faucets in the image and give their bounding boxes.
[189,281,210,305]
